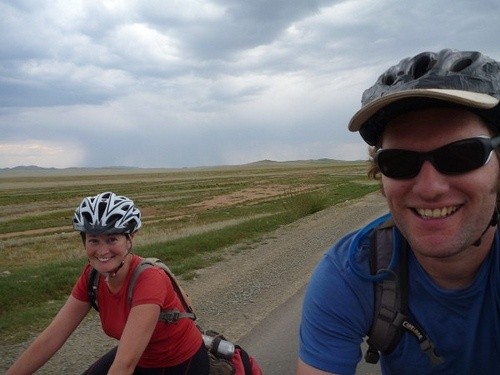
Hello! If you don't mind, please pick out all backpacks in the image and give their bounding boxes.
[87,257,197,324]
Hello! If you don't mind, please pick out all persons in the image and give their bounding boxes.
[297,50,500,375]
[7,192,211,375]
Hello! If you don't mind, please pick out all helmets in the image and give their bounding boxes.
[347,48,500,147]
[72,192,141,235]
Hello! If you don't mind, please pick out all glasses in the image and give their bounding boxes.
[370,134,500,181]
